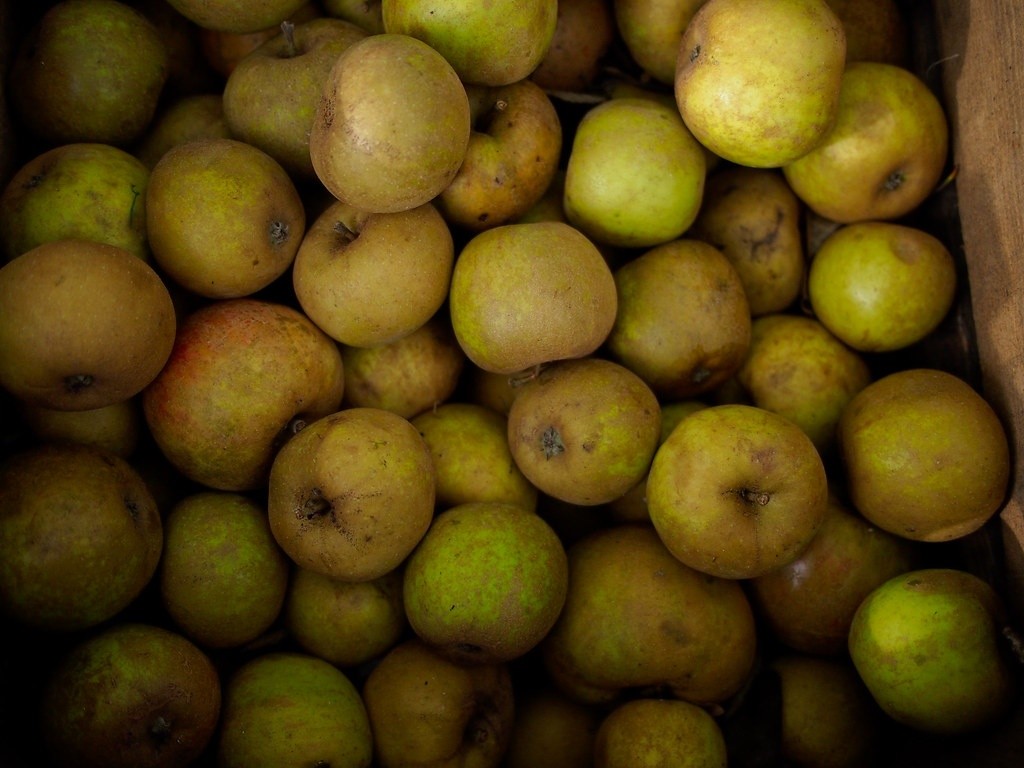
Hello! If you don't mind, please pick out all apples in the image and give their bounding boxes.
[0,0,1018,768]
